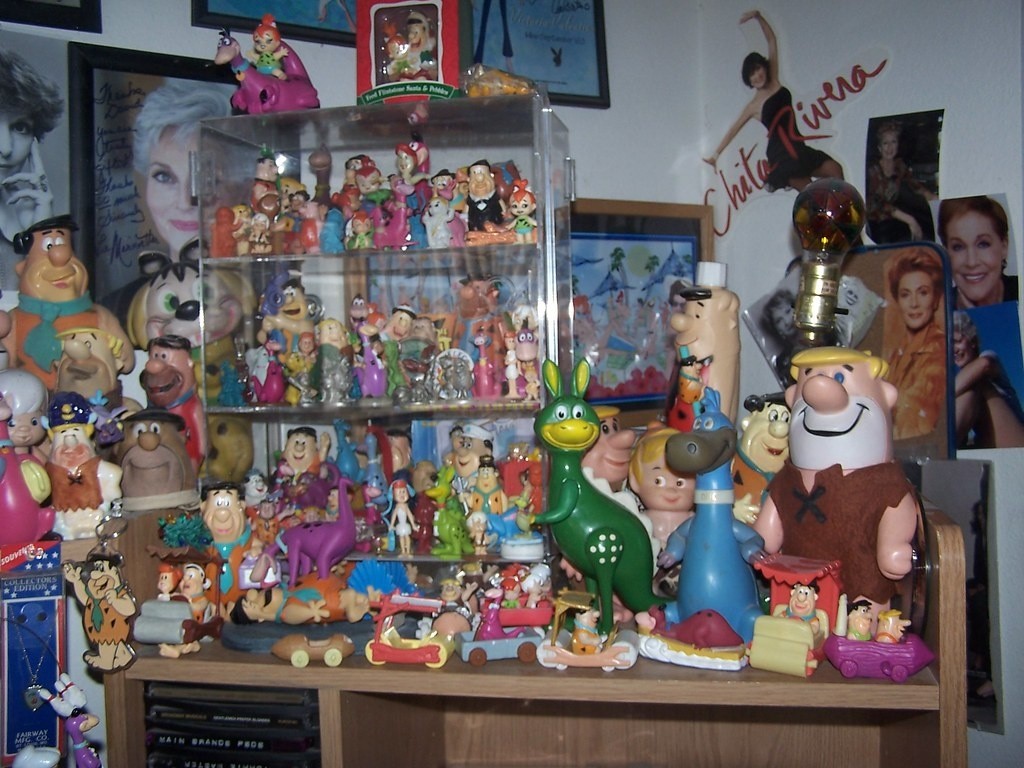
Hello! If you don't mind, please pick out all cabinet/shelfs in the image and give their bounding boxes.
[97,510,970,768]
[202,241,547,570]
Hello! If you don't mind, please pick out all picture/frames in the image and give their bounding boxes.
[190,1,612,111]
[65,42,253,330]
[1,0,103,33]
[343,195,715,430]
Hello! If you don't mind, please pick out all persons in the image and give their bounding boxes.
[867,118,935,245]
[759,289,820,383]
[949,310,1023,448]
[885,247,946,437]
[939,197,1019,306]
[0,49,66,288]
[703,10,844,193]
[96,78,237,331]
